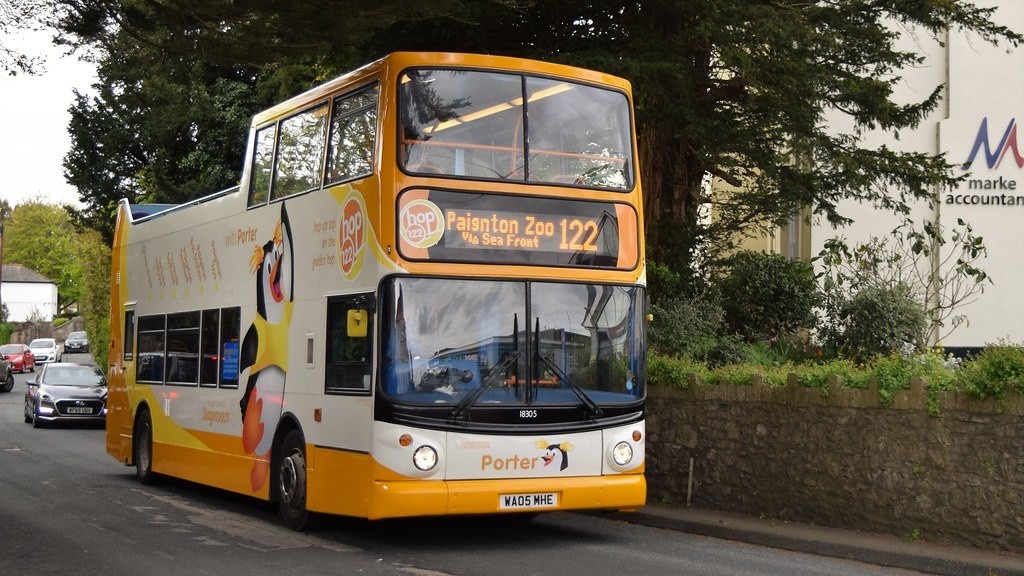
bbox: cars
[0,343,36,373]
[0,352,15,393]
[24,362,108,429]
[64,331,92,352]
[28,337,61,363]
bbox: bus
[105,49,655,533]
